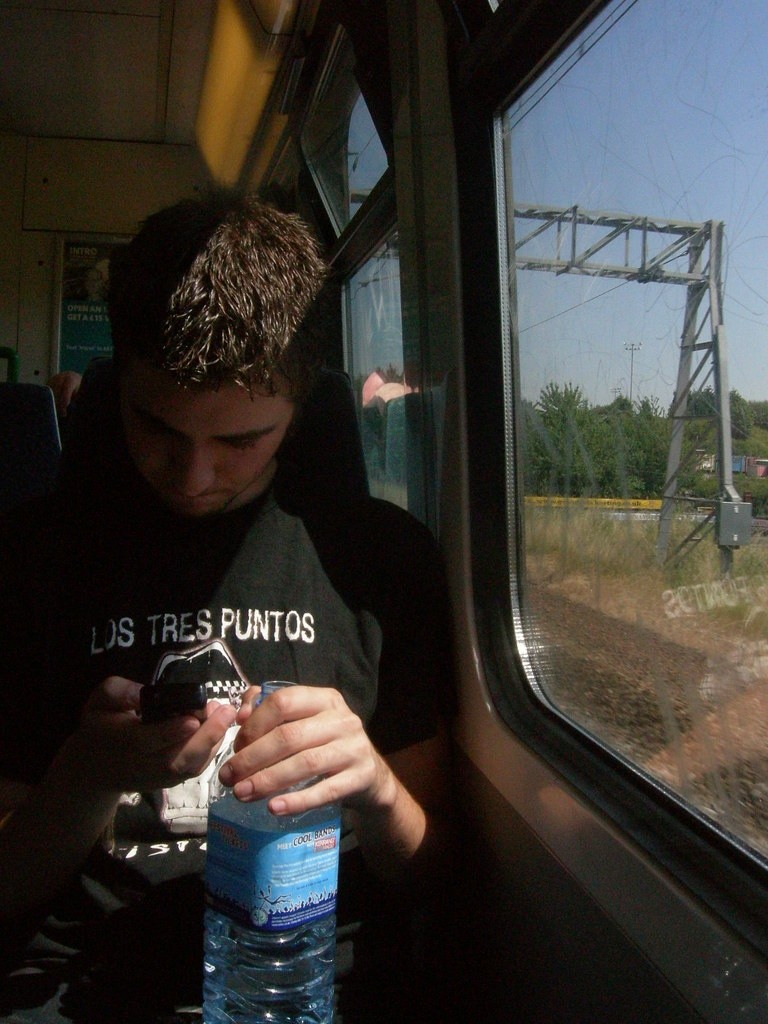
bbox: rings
[50,383,62,390]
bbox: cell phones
[139,681,208,728]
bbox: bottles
[202,680,343,1024]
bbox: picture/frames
[49,232,131,378]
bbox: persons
[45,370,82,416]
[0,185,459,1024]
